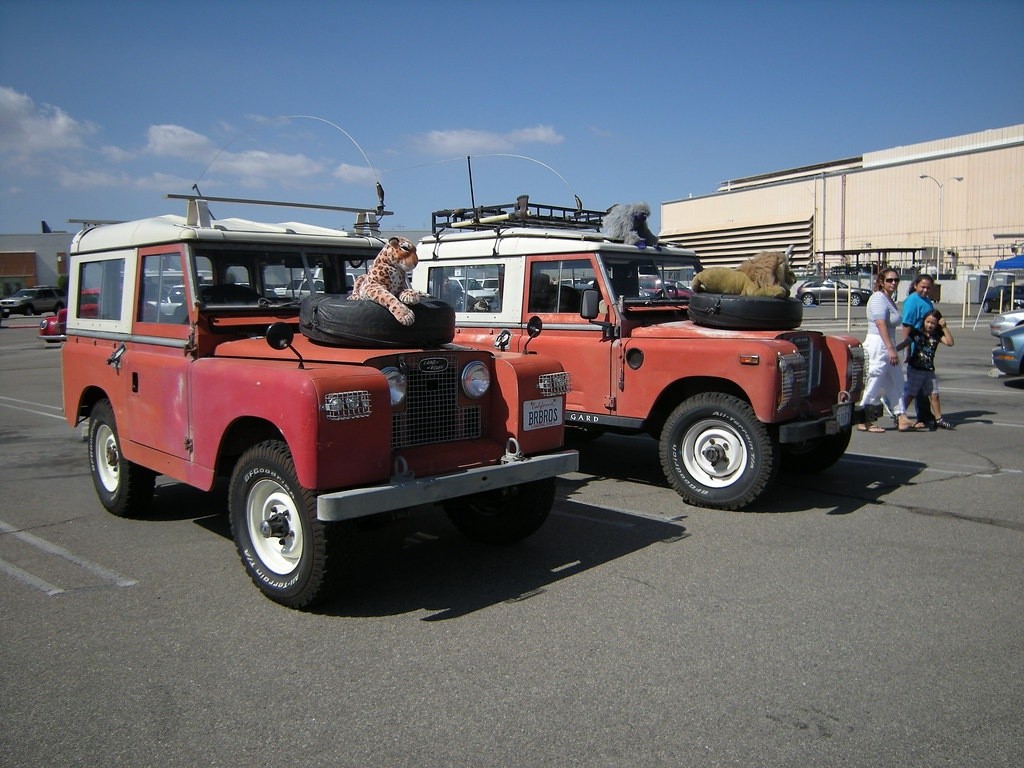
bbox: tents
[973,254,1024,332]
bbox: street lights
[920,174,964,280]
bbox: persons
[856,269,927,433]
[901,274,935,425]
[442,276,456,305]
[895,310,957,430]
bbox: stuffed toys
[690,252,799,298]
[602,201,660,249]
[346,235,420,325]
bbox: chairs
[532,273,578,314]
[200,282,260,305]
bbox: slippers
[858,423,885,433]
[900,421,926,432]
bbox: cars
[983,284,1024,313]
[116,265,690,317]
[992,322,1024,377]
[990,308,1024,338]
[39,287,101,345]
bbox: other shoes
[917,416,938,427]
[880,395,895,418]
[936,419,956,429]
[893,413,901,426]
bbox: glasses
[885,278,900,283]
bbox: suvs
[0,284,67,319]
[62,193,580,611]
[409,194,865,513]
[796,278,873,307]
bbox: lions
[690,251,797,298]
